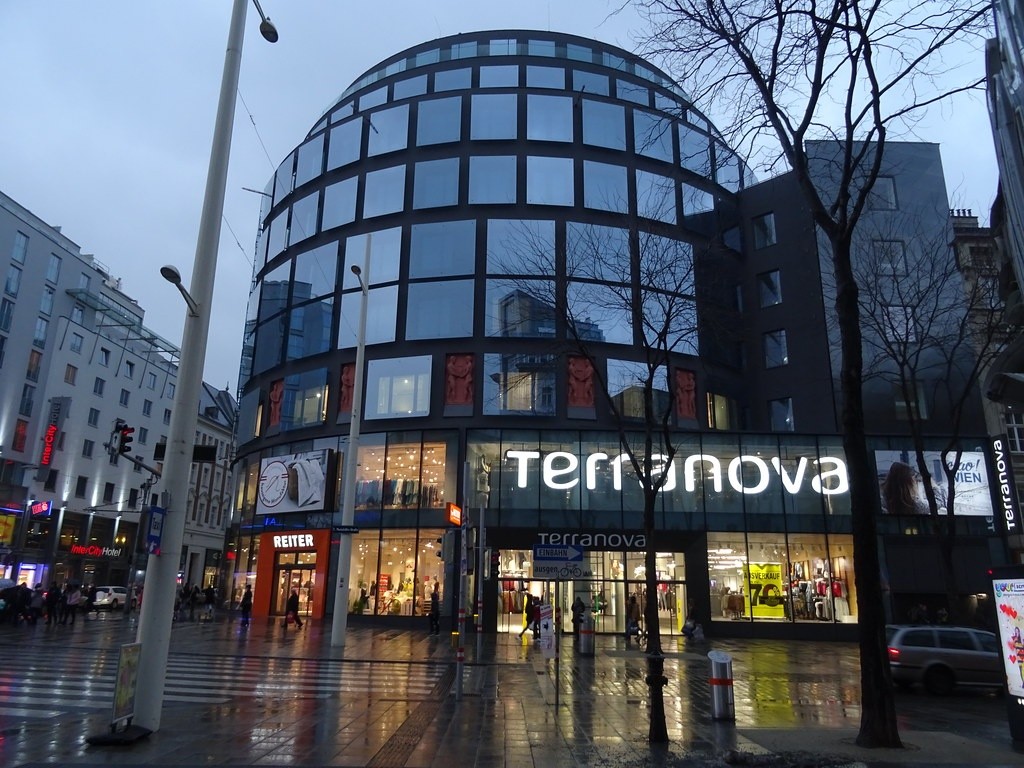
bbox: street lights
[108,261,214,732]
[329,264,372,646]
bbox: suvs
[90,586,138,610]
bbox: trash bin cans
[707,648,738,721]
[578,621,596,655]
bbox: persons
[135,585,144,628]
[370,580,376,595]
[7,580,101,626]
[519,592,546,640]
[446,355,473,404]
[178,583,200,621]
[353,582,370,615]
[414,575,432,601]
[280,588,303,628]
[269,381,283,426]
[572,596,585,634]
[239,585,252,627]
[681,598,699,641]
[432,574,440,600]
[427,593,441,637]
[880,461,930,514]
[341,366,354,411]
[624,596,648,646]
[1012,626,1024,688]
[719,587,730,600]
[676,370,696,418]
[568,356,595,406]
[204,584,216,616]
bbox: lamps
[118,511,123,517]
[30,494,35,500]
[62,501,68,508]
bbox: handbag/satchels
[692,623,705,640]
[629,622,638,635]
[681,618,696,639]
[286,612,295,623]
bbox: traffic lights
[108,414,135,456]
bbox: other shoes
[435,634,441,636]
[519,634,522,640]
[428,633,435,636]
[298,623,303,628]
[45,620,74,625]
[281,625,287,628]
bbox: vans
[883,623,1007,702]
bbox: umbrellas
[0,578,16,589]
